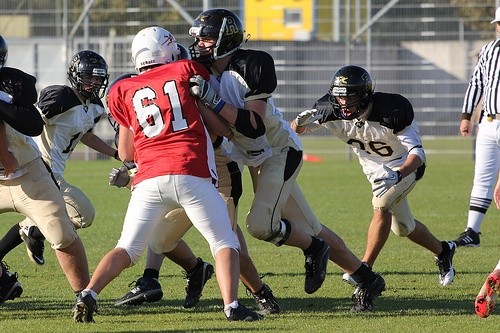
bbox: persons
[0,50,121,300]
[107,73,213,311]
[448,6,500,246]
[290,66,457,287]
[475,172,500,317]
[73,27,265,322]
[149,44,279,315]
[188,8,386,311]
[0,34,91,307]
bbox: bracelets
[114,151,118,158]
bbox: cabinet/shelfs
[73,44,467,136]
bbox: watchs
[392,167,402,184]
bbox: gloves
[108,166,131,188]
[190,74,222,108]
[296,108,322,127]
[371,163,402,197]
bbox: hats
[490,7,500,24]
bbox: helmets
[131,26,178,71]
[0,35,7,69]
[68,51,109,100]
[328,64,373,120]
[187,8,245,67]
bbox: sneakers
[475,270,500,318]
[223,301,264,322]
[304,238,330,294]
[342,273,358,285]
[243,282,279,314]
[0,271,23,305]
[73,291,98,323]
[434,240,457,286]
[19,225,45,265]
[452,226,481,246]
[182,257,215,308]
[350,273,385,313]
[115,277,163,306]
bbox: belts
[488,113,500,120]
